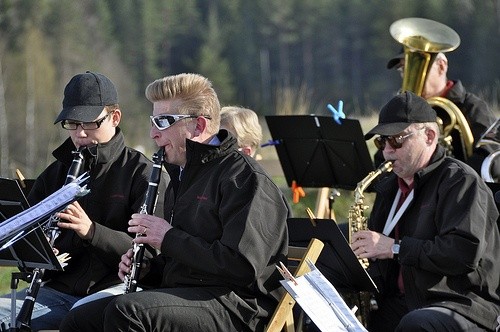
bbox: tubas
[389,16,474,161]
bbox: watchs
[392,238,401,261]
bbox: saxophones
[347,160,394,330]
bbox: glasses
[61,107,117,130]
[374,126,426,150]
[150,115,212,130]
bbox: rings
[143,227,146,233]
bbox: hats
[54,71,118,125]
[387,47,448,70]
[365,91,437,140]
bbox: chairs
[265,238,324,332]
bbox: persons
[70,105,262,311]
[349,88,500,332]
[386,44,500,223]
[0,66,171,332]
[61,73,289,332]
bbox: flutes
[8,146,86,330]
[124,145,165,294]
